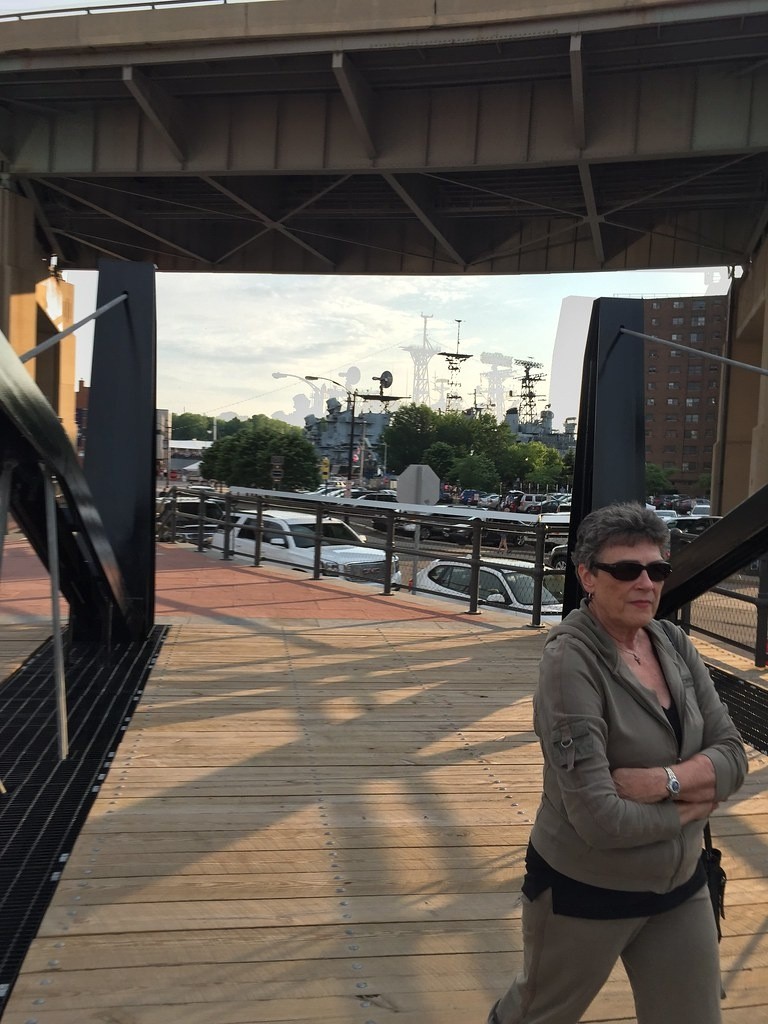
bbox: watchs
[664,766,681,802]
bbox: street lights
[305,375,357,499]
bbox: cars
[295,480,725,572]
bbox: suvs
[210,509,403,591]
[407,553,565,628]
[154,496,224,549]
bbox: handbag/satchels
[702,846,726,944]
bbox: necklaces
[616,631,642,666]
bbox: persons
[487,504,749,1024]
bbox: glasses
[591,560,673,583]
